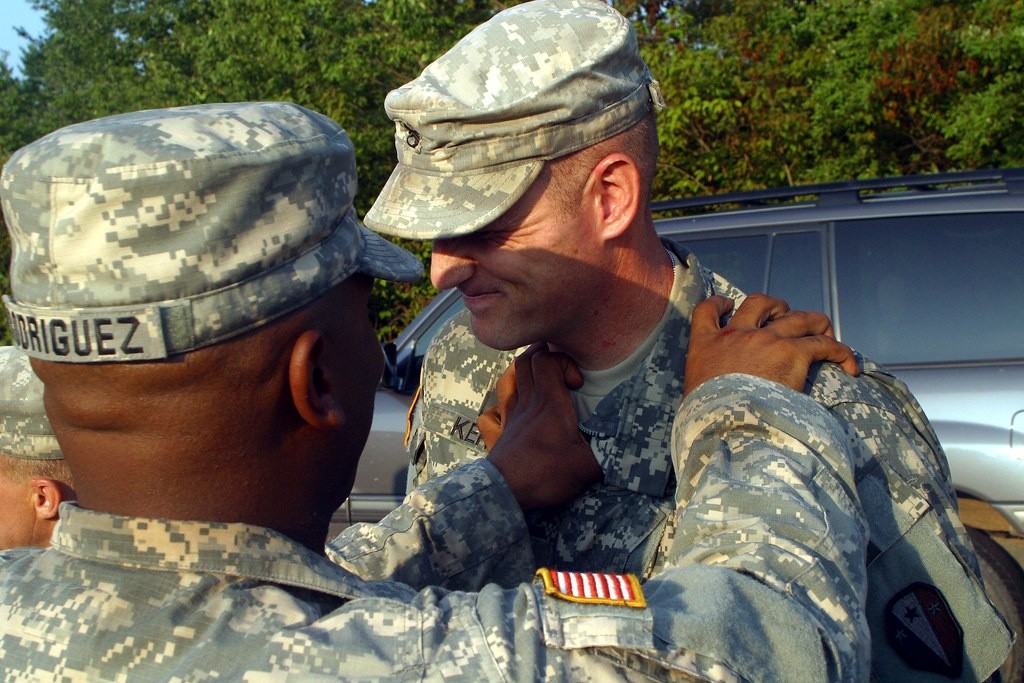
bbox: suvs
[322,170,1024,683]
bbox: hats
[0,346,65,460]
[364,0,666,240]
[0,101,424,363]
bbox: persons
[364,1,1017,683]
[0,102,871,683]
[0,344,77,550]
